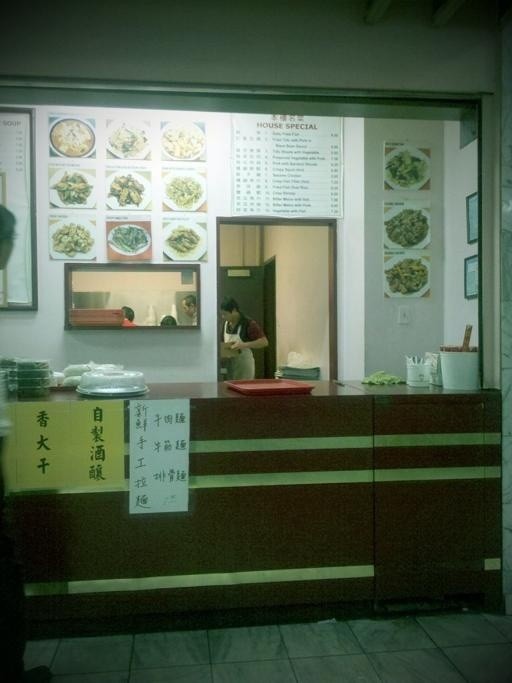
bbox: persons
[159,316,177,325]
[120,306,136,326]
[220,295,269,381]
[183,294,197,327]
[0,205,54,682]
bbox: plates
[48,116,207,261]
[383,146,433,298]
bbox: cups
[408,364,430,388]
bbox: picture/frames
[0,105,38,310]
[463,253,478,300]
[466,191,478,245]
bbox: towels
[362,371,406,385]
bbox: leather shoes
[24,666,52,683]
